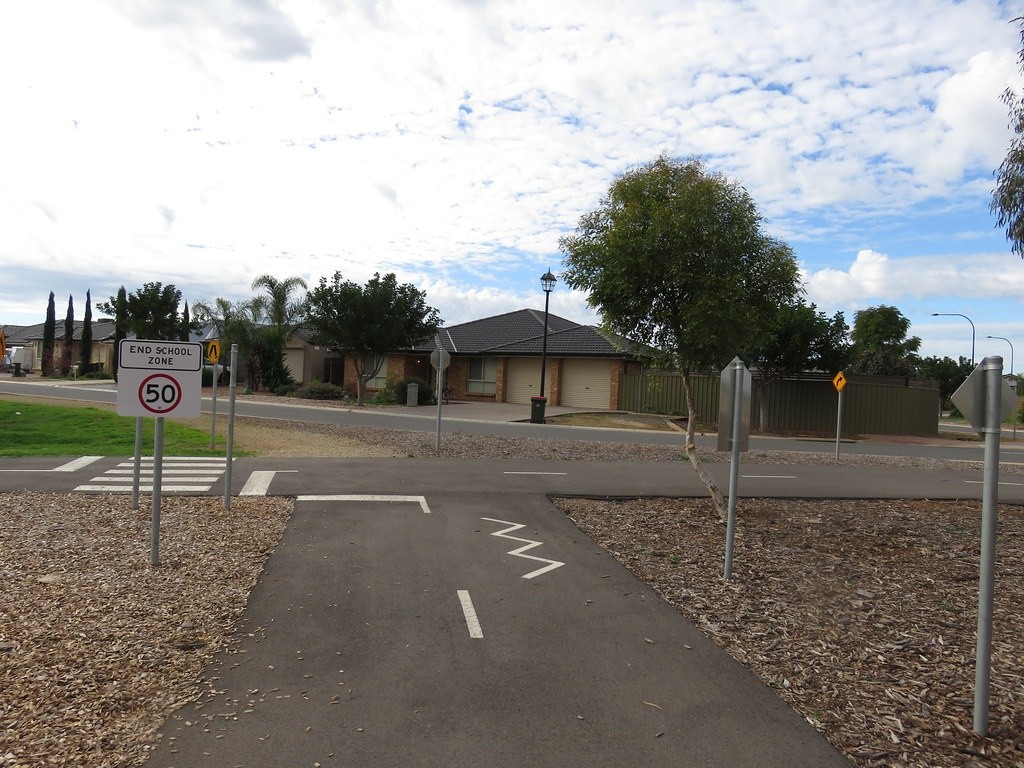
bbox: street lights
[987,335,1014,381]
[930,313,976,369]
[539,267,557,397]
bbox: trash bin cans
[530,396,547,424]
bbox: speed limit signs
[116,338,203,419]
